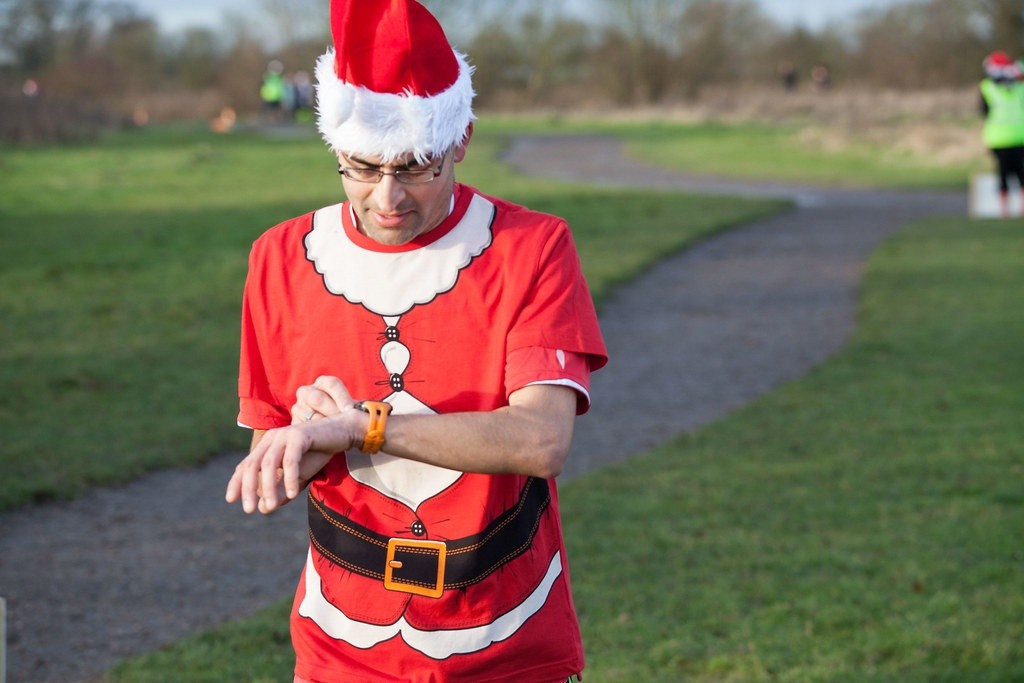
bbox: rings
[306,410,316,420]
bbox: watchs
[354,399,393,456]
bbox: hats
[982,52,1019,80]
[315,0,477,165]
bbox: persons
[222,0,610,682]
[256,56,316,124]
[976,50,1024,218]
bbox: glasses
[338,146,447,185]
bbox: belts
[307,476,552,598]
